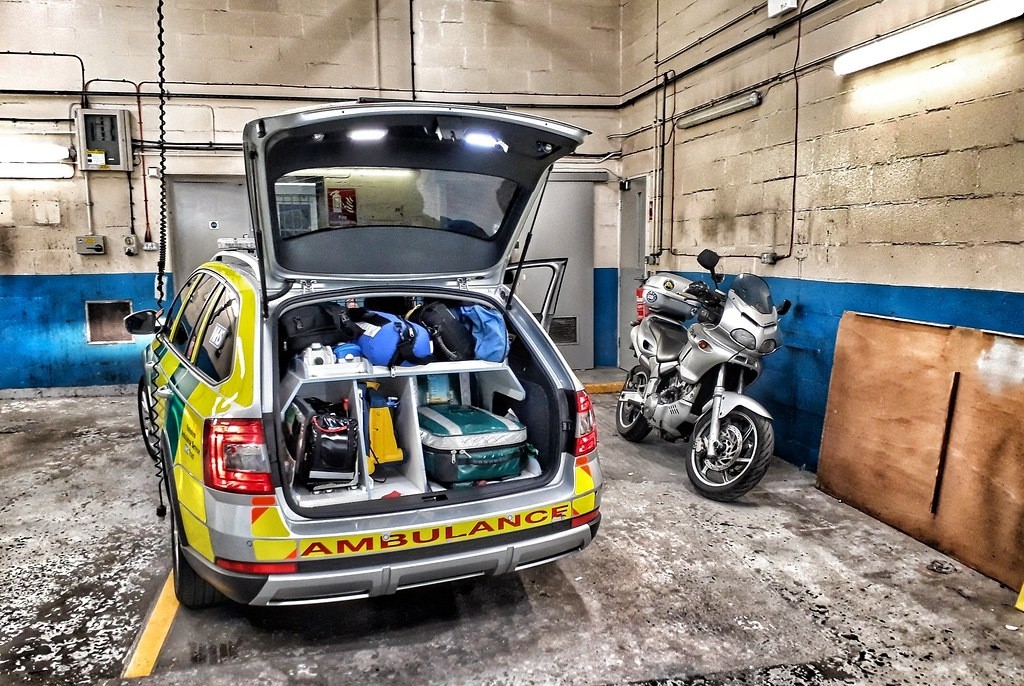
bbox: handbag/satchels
[278,301,511,378]
[303,413,359,492]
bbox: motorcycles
[616,249,792,502]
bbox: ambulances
[124,97,604,608]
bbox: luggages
[417,404,527,489]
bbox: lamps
[832,0,1024,78]
[675,89,763,129]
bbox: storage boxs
[645,273,699,321]
